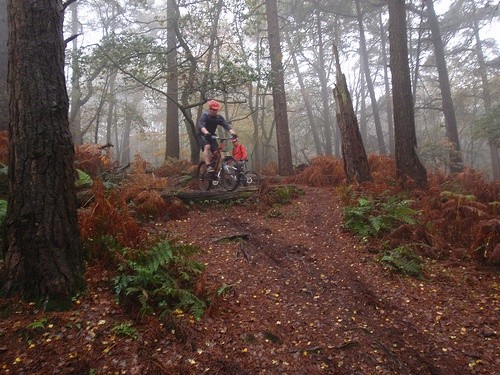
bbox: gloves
[204,133,212,142]
[231,133,238,140]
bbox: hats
[208,100,220,109]
[231,139,237,143]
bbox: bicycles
[218,157,260,187]
[195,135,241,191]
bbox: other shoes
[206,166,215,174]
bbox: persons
[195,99,238,178]
[232,138,248,171]
